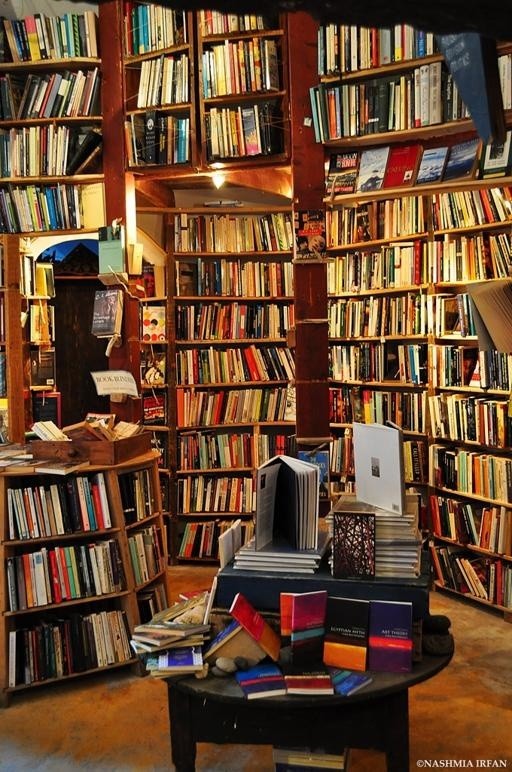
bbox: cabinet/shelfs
[1,244,62,445]
[0,449,172,706]
[0,0,106,234]
[140,207,296,565]
[121,2,292,172]
[312,24,512,620]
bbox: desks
[163,603,454,772]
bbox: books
[186,739,398,769]
[315,204,504,455]
[131,580,435,709]
[11,177,112,230]
[191,554,331,584]
[172,202,301,552]
[6,8,512,195]
[4,465,175,690]
[257,444,324,550]
[315,453,421,571]
[2,234,170,460]
[428,449,509,612]
